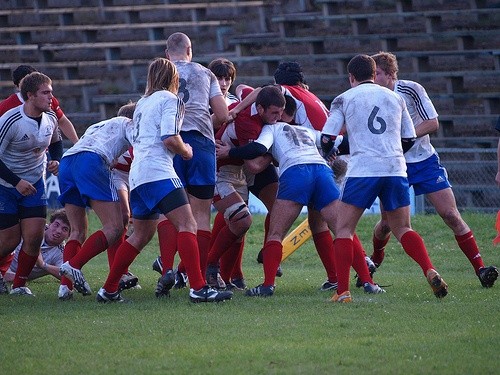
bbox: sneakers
[126,272,141,289]
[319,280,338,292]
[96,287,134,305]
[189,284,233,302]
[246,283,276,296]
[0,272,11,294]
[329,291,351,303]
[58,261,92,296]
[118,274,138,292]
[206,265,247,291]
[58,284,73,300]
[426,269,448,298]
[353,256,376,286]
[9,283,35,297]
[363,283,385,296]
[152,256,163,276]
[154,268,176,300]
[476,266,499,288]
[256,247,282,277]
[172,271,188,289]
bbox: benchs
[1,0,500,212]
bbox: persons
[321,55,448,301]
[356,51,498,288]
[494,138,500,185]
[0,32,386,301]
